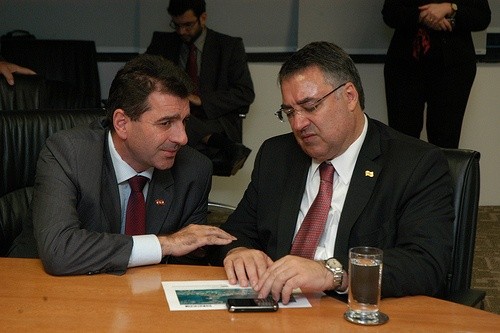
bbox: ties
[187,44,201,93]
[125,175,148,236]
[289,165,336,259]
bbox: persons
[378,0,492,153]
[6,53,239,279]
[198,41,458,307]
[0,61,38,87]
[157,0,255,158]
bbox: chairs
[0,34,104,261]
[431,147,489,313]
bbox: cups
[345,247,383,325]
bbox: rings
[430,16,433,22]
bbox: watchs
[322,257,344,291]
[450,2,458,15]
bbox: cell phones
[226,298,278,312]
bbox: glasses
[275,82,348,123]
[168,15,203,30]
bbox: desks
[1,253,500,333]
